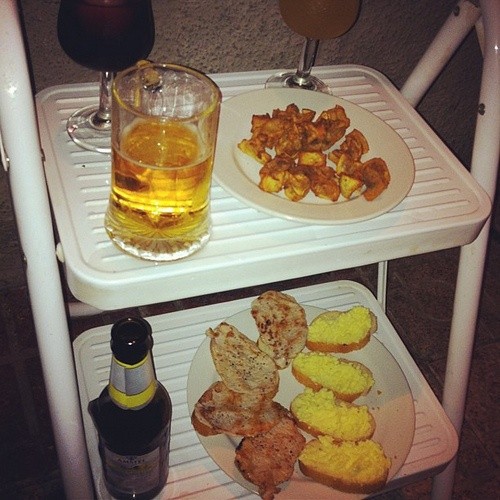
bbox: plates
[209,88,416,224]
[188,306,416,499]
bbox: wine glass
[57,1,155,154]
[265,0,360,98]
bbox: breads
[292,351,374,403]
[250,289,309,369]
[289,393,377,440]
[205,321,281,395]
[299,437,391,493]
[191,381,287,438]
[233,409,306,500]
[304,305,378,353]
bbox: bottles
[95,317,172,500]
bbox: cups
[105,59,222,261]
